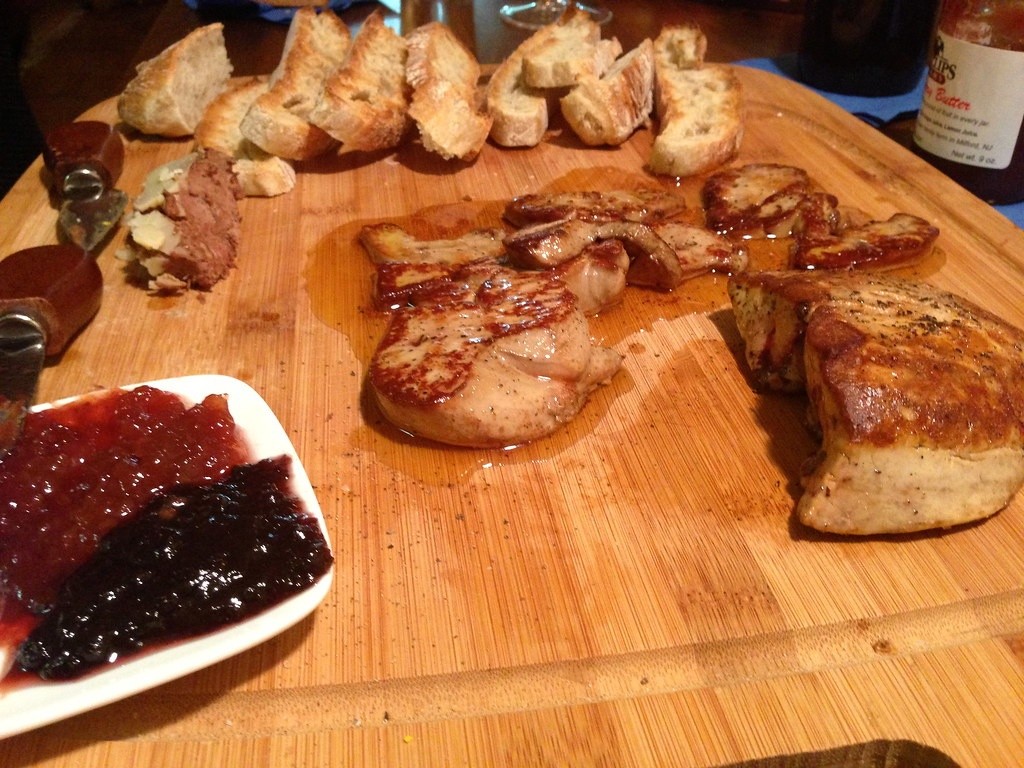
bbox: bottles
[914,1,1024,204]
[799,0,936,97]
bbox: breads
[113,7,744,196]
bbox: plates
[1,375,335,739]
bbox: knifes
[0,338,45,476]
[43,121,129,251]
[1,245,104,359]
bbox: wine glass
[501,0,615,30]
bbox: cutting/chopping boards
[1,62,1024,766]
